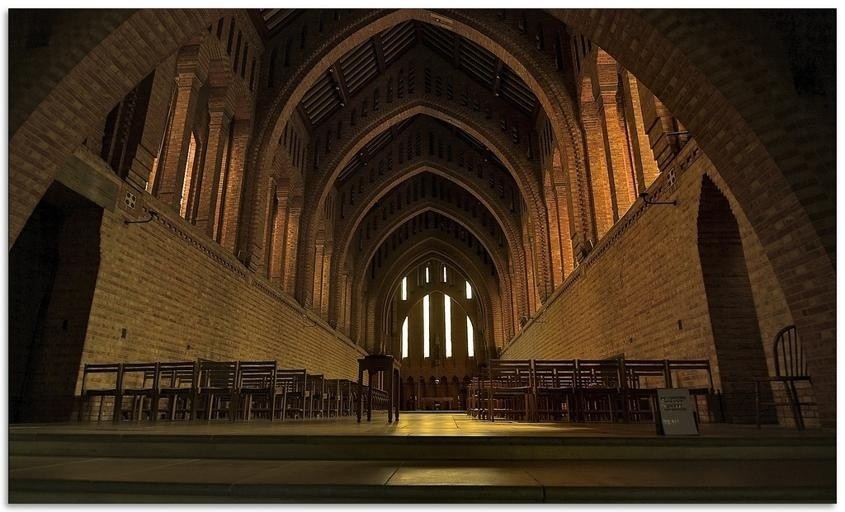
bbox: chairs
[754,324,812,431]
[466,354,722,423]
